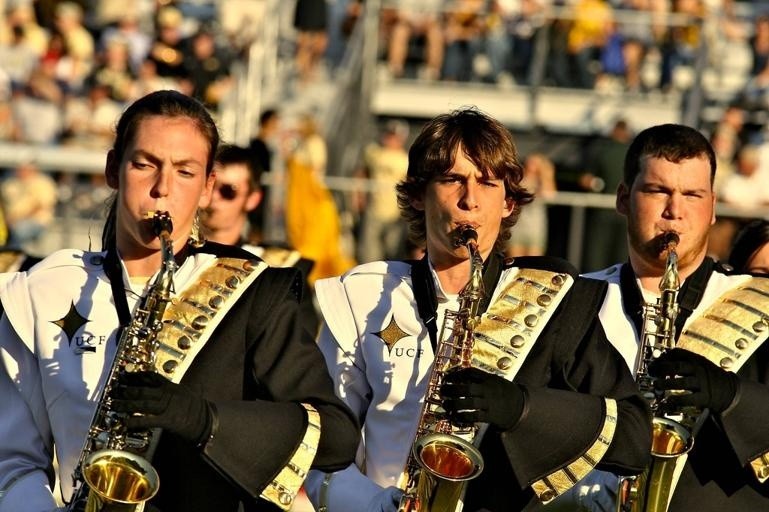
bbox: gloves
[108,369,211,446]
[648,347,738,414]
[440,367,526,431]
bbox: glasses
[208,181,235,201]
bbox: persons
[1,91,358,512]
[1,0,768,340]
[301,111,654,511]
[570,124,768,511]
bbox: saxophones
[618,229,694,512]
[399,224,485,512]
[67,209,179,512]
